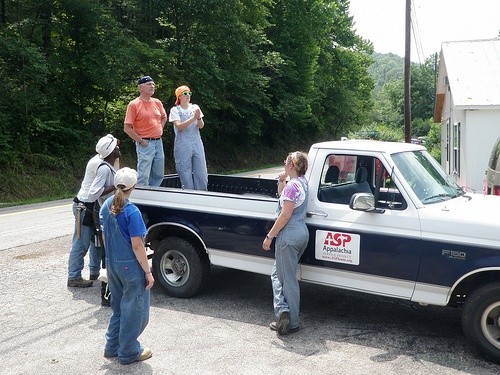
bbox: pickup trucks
[328,154,390,188]
[128,140,500,364]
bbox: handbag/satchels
[101,281,112,306]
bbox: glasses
[181,92,192,96]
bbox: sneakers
[135,346,153,361]
[90,273,99,280]
[68,277,93,287]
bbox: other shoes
[269,321,299,332]
[277,311,290,336]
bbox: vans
[483,136,500,195]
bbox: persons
[123,76,167,187]
[263,150,309,336]
[168,85,208,192]
[99,167,155,364]
[88,134,122,306]
[66,155,119,288]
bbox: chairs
[320,165,372,205]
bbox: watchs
[266,236,273,240]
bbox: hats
[136,75,153,87]
[114,167,138,190]
[175,86,190,105]
[96,134,117,158]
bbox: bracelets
[197,117,202,120]
[145,271,151,274]
[138,139,143,144]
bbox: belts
[142,137,160,140]
